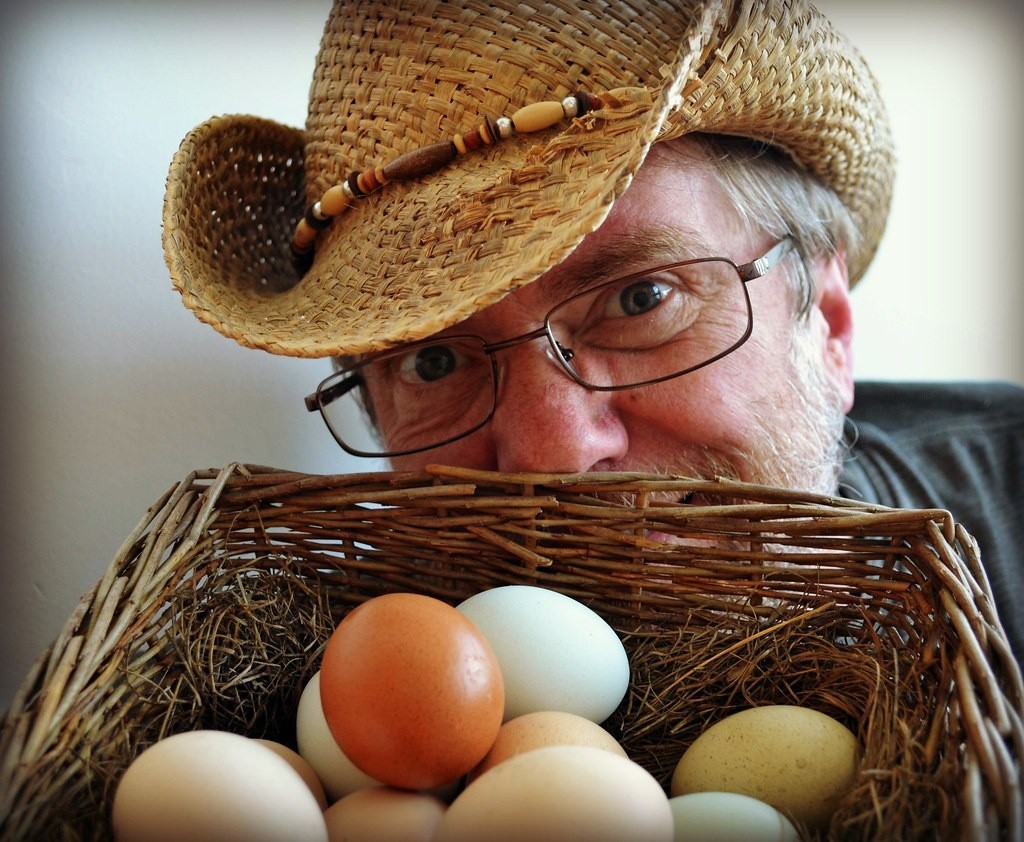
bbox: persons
[162,0,1024,674]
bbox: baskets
[0,461,1024,842]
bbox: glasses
[303,232,799,458]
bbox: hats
[161,0,898,359]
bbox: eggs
[112,584,858,842]
